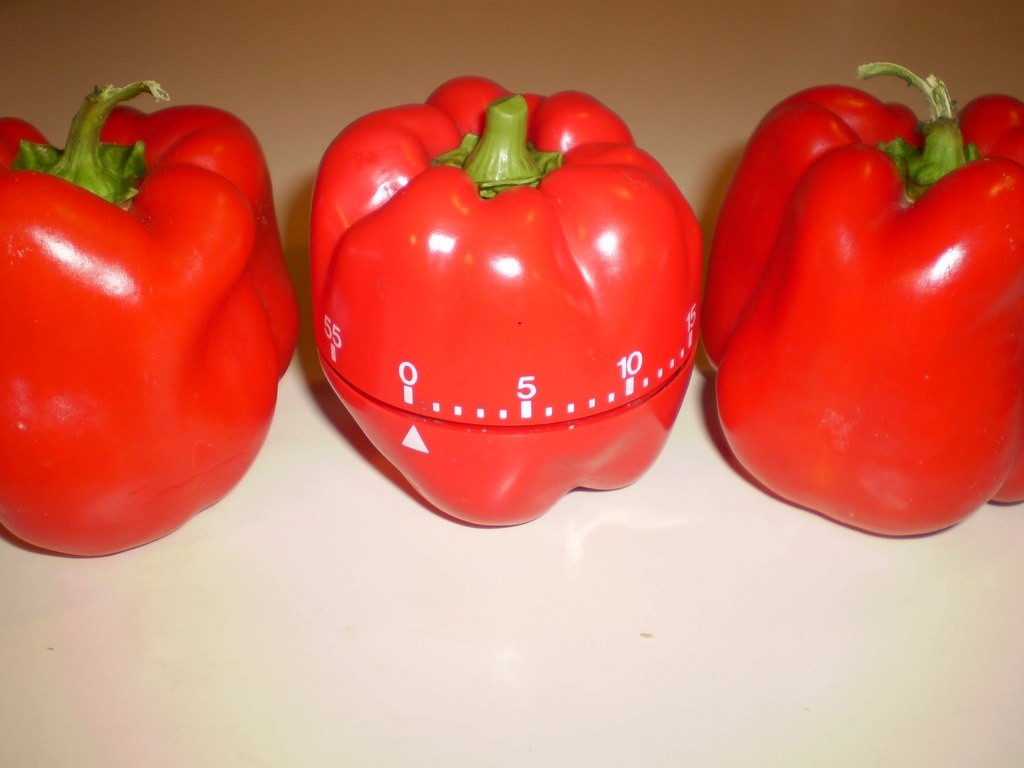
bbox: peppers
[309,75,703,527]
[1,79,301,555]
[703,60,1024,536]
